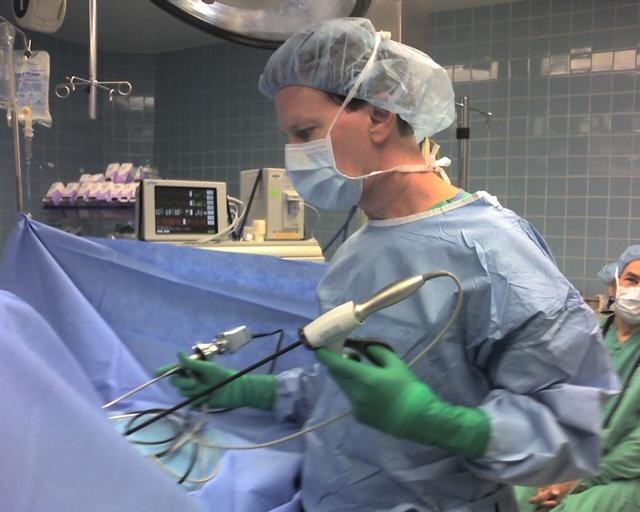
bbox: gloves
[314,343,489,461]
[151,350,276,413]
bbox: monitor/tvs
[136,177,226,242]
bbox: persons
[500,239,640,512]
[150,15,625,512]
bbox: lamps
[149,0,372,51]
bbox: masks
[613,277,640,327]
[282,97,417,212]
